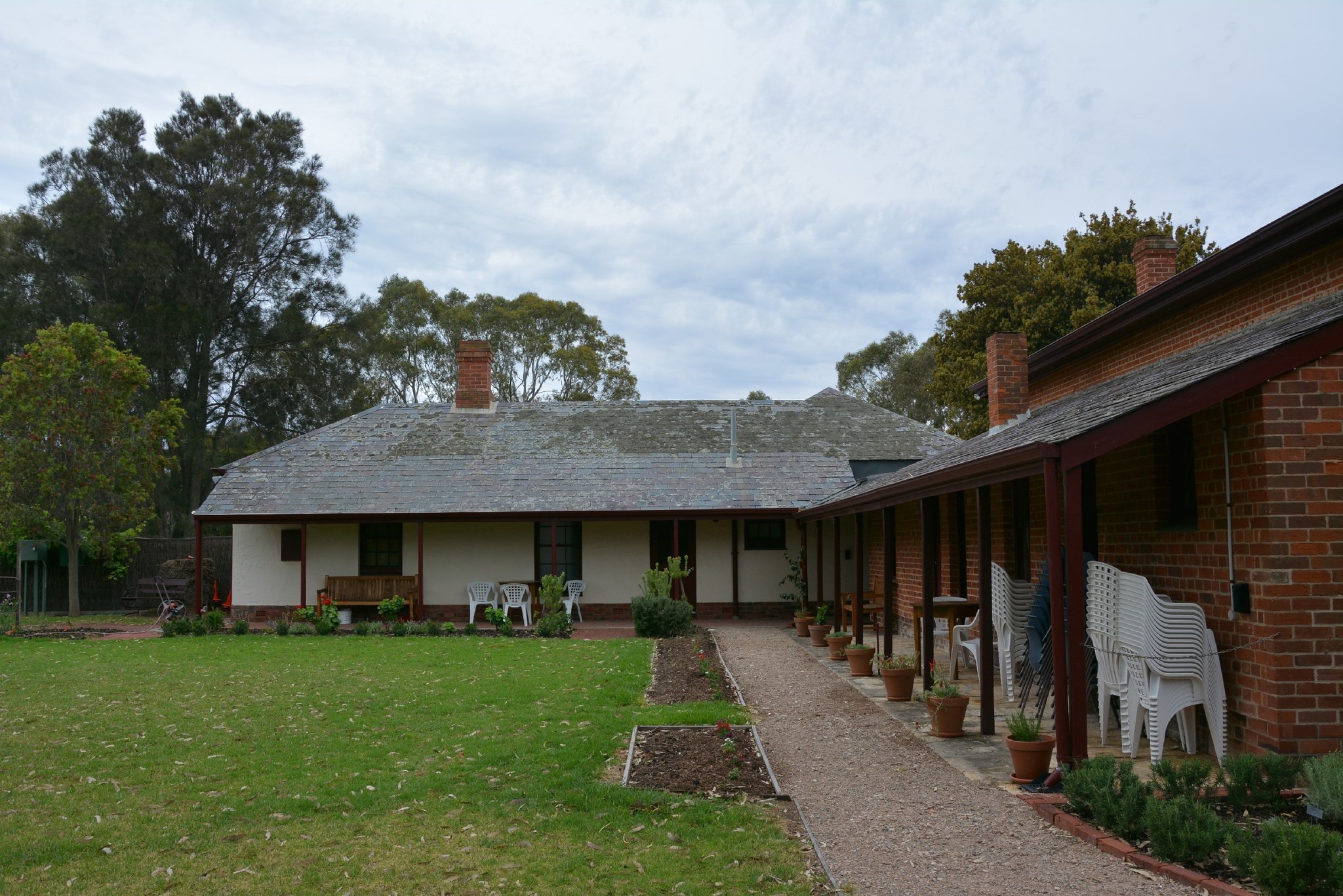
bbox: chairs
[559,580,586,622]
[921,547,1229,770]
[500,584,532,627]
[468,581,498,625]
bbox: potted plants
[1001,708,1056,782]
[808,604,832,646]
[834,642,876,676]
[824,631,852,659]
[778,546,815,636]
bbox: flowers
[911,658,968,736]
[869,650,920,671]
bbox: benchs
[839,575,896,635]
[317,574,418,622]
[121,577,188,614]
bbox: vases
[925,694,969,736]
[879,666,914,701]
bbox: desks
[498,580,542,624]
[909,600,980,680]
